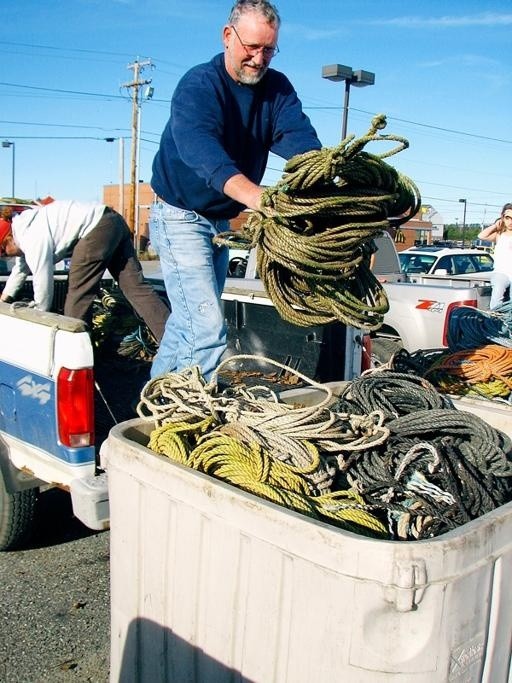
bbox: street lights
[2,139,17,203]
[321,63,378,150]
[459,198,467,249]
[104,135,125,230]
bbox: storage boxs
[97,379,512,683]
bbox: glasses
[231,26,279,58]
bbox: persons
[0,199,171,348]
[475,202,511,309]
[147,0,322,384]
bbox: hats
[0,218,11,256]
